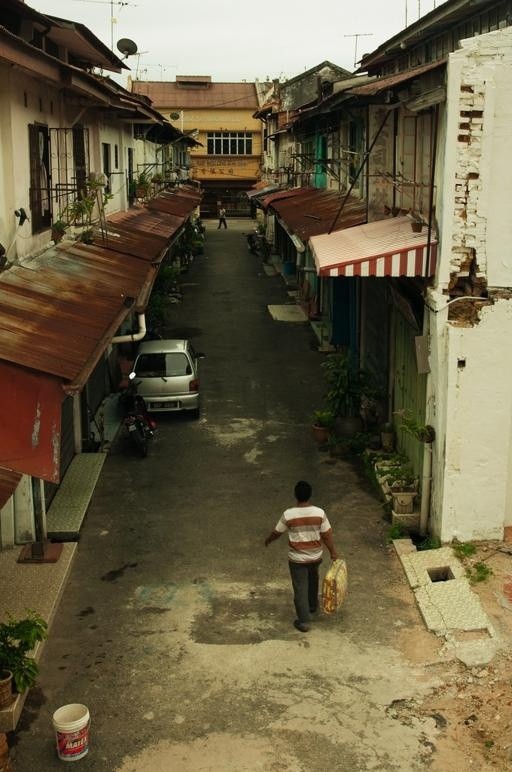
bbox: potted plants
[310,345,421,514]
[134,172,153,197]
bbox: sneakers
[293,619,310,631]
[310,599,319,611]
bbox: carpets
[119,360,134,386]
[269,305,310,323]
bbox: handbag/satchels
[322,558,348,614]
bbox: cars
[134,340,207,420]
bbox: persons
[216,205,228,230]
[263,480,339,634]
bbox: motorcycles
[116,371,158,460]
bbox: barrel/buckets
[53,703,91,761]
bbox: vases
[0,669,14,707]
